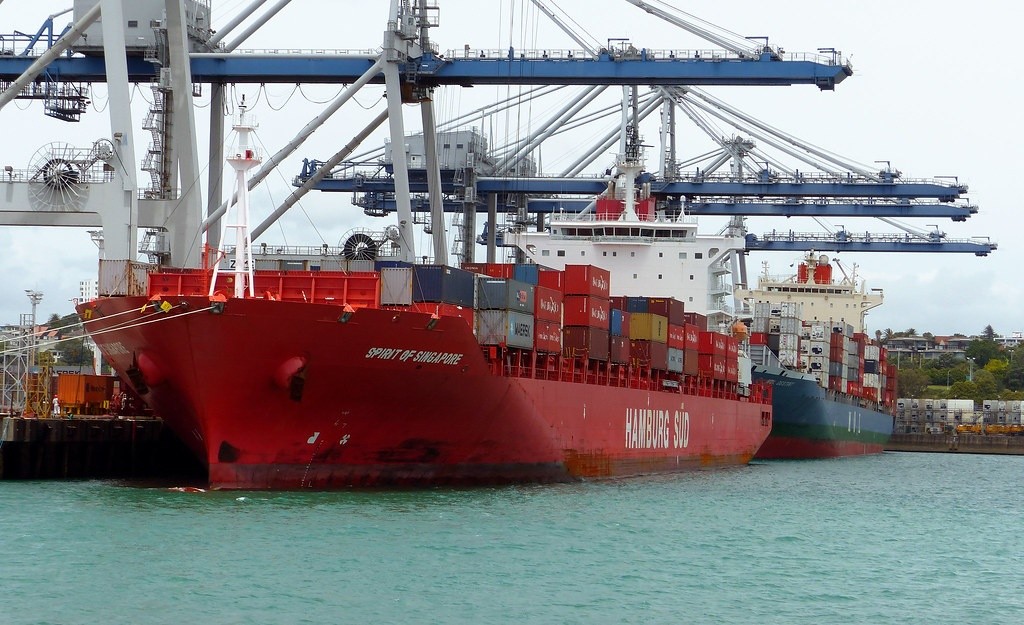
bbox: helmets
[54,395,57,397]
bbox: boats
[0,0,1009,495]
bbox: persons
[52,394,60,414]
